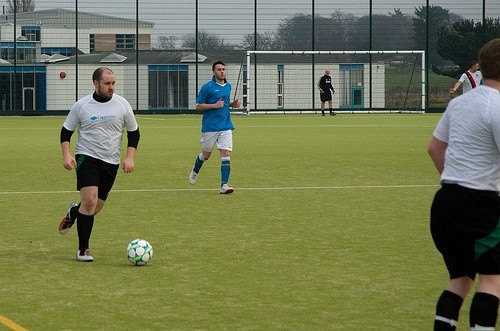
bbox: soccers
[127,239,152,266]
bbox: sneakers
[189,165,199,185]
[219,183,234,194]
[76,249,94,262]
[58,201,78,235]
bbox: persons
[189,60,240,194]
[449,60,483,95]
[58,67,141,262]
[427,36,500,331]
[317,69,336,116]
